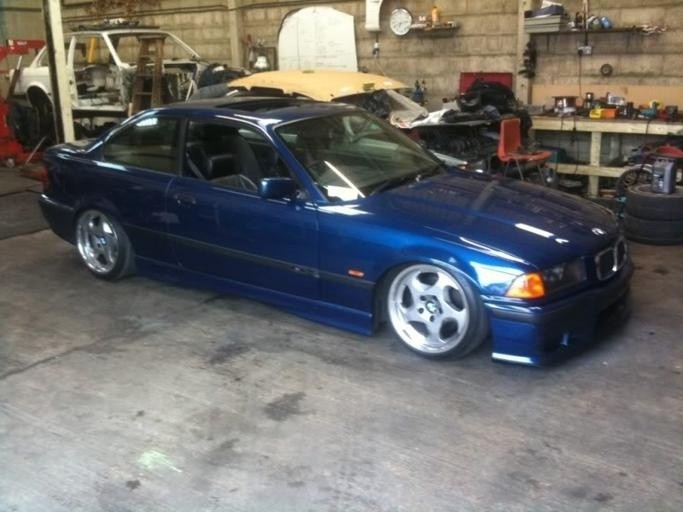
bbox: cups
[625,102,633,120]
[665,106,678,122]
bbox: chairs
[205,153,258,194]
[494,115,555,188]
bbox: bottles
[430,6,439,23]
[586,93,592,109]
[588,14,611,30]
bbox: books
[524,15,570,33]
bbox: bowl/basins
[550,95,578,108]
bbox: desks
[518,111,682,200]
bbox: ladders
[131,33,169,116]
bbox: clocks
[388,6,413,37]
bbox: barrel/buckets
[651,157,677,194]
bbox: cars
[35,92,634,369]
[3,18,251,145]
[187,67,557,193]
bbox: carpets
[0,189,51,241]
